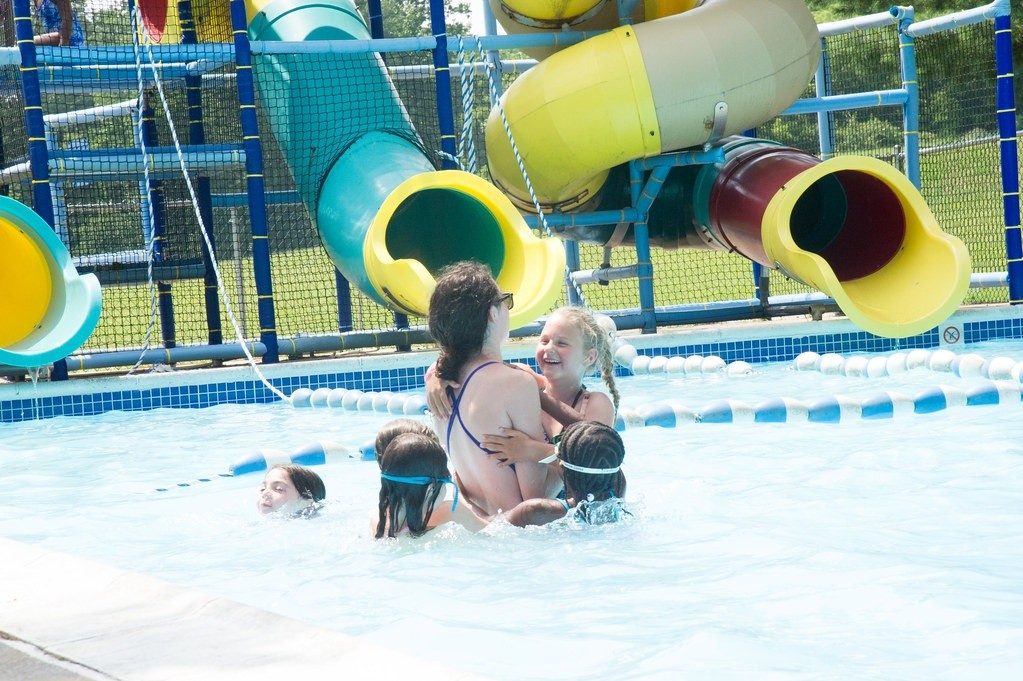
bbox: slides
[1,195,100,365]
[242,0,971,339]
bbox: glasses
[492,292,514,310]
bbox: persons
[423,305,621,500]
[374,418,491,535]
[252,465,325,521]
[374,429,451,539]
[452,420,637,534]
[422,257,569,523]
